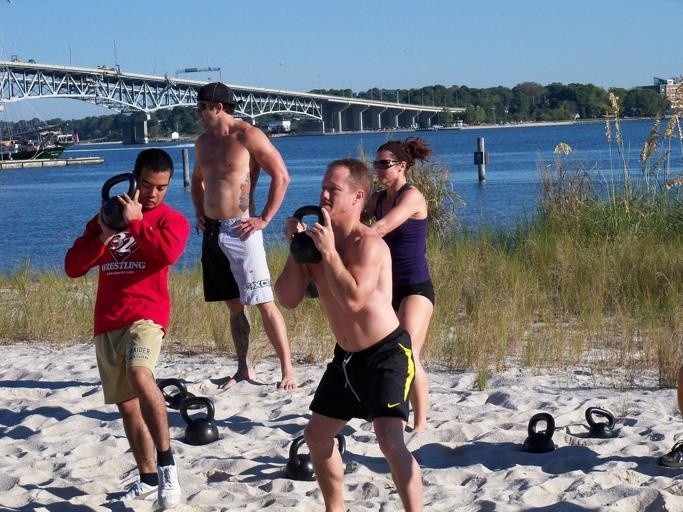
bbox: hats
[197,82,237,104]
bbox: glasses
[372,160,401,169]
[196,102,214,111]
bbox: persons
[273,158,423,511]
[64,148,190,510]
[304,138,436,434]
[191,81,297,392]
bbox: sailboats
[0,47,75,162]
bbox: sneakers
[157,459,181,511]
[122,483,159,502]
[657,443,683,467]
